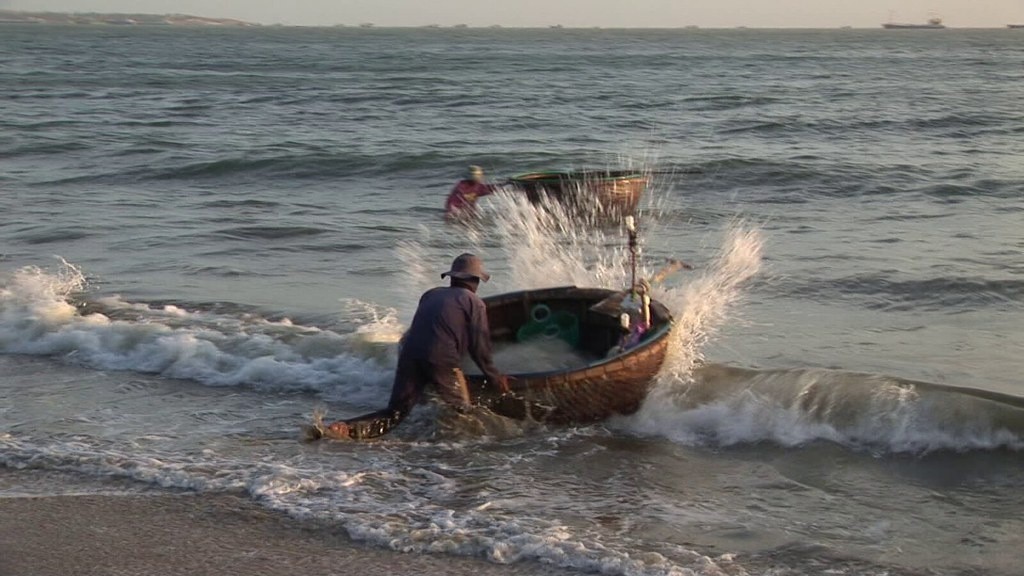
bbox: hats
[441,252,489,284]
[462,165,485,180]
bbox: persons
[441,165,508,226]
[328,254,510,441]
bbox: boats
[506,168,650,222]
[397,285,674,424]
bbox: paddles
[635,166,705,174]
[585,258,693,320]
